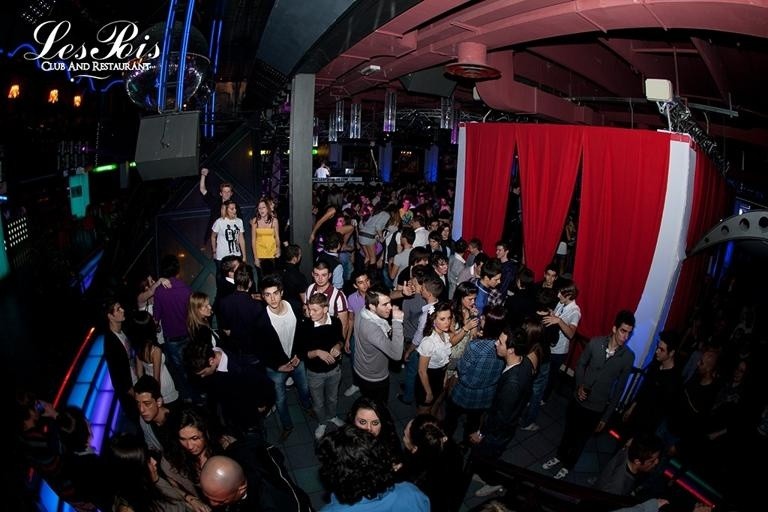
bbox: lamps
[349,103,362,139]
[382,90,398,133]
[328,113,338,142]
[335,99,344,132]
[645,78,731,175]
[450,119,460,145]
[440,98,453,129]
[312,116,319,148]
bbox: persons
[9,159,767,512]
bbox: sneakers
[279,426,295,443]
[343,384,361,397]
[314,423,327,441]
[520,423,539,430]
[471,473,502,497]
[541,457,569,480]
[396,381,414,407]
[328,416,344,427]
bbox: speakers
[400,66,458,98]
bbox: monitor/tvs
[344,168,354,177]
